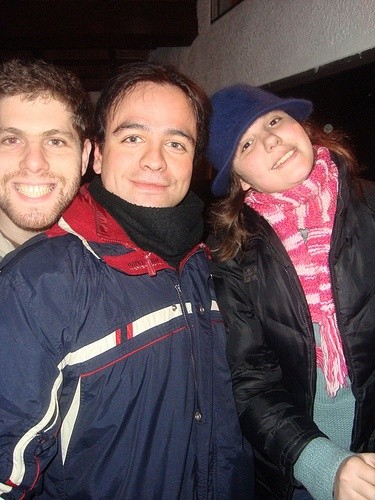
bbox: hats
[206,84,312,197]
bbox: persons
[203,81,375,500]
[0,65,253,499]
[0,59,91,263]
